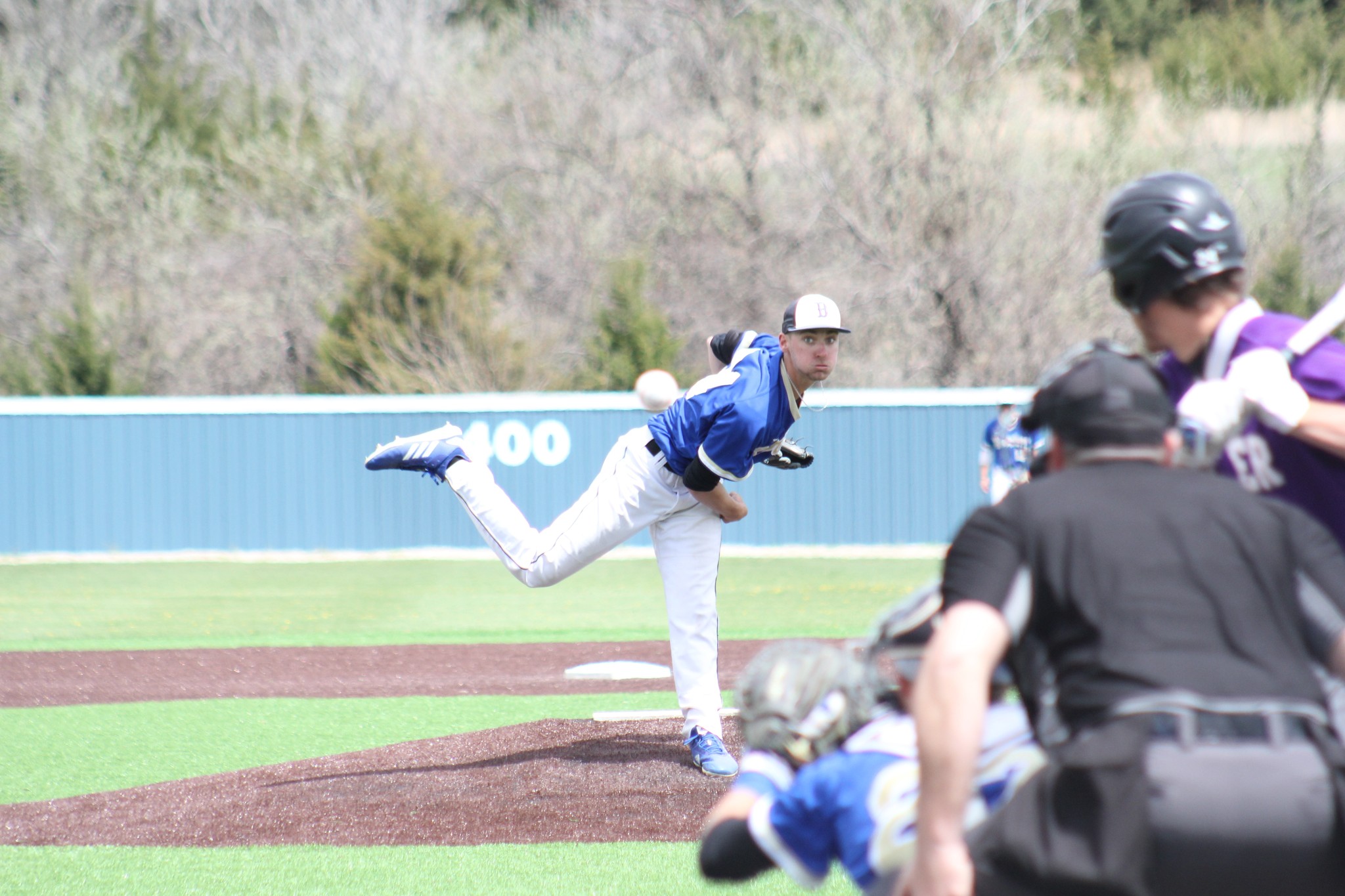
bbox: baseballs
[632,366,680,412]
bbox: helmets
[1102,173,1245,308]
[867,587,1014,684]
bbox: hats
[1020,339,1175,434]
[782,293,851,336]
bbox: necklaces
[781,357,828,412]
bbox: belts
[1102,710,1312,740]
[647,440,681,477]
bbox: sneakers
[689,726,738,777]
[364,425,472,483]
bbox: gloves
[1176,379,1250,463]
[1224,347,1307,433]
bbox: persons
[367,295,851,778]
[699,174,1345,896]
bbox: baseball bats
[1178,283,1345,458]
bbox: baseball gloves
[760,435,815,470]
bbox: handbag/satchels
[969,722,1151,896]
